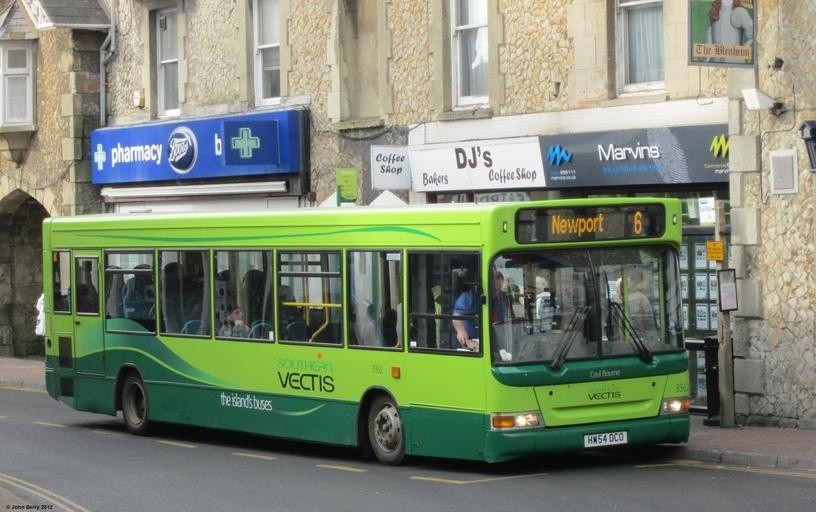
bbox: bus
[42,197,690,465]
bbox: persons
[216,303,252,339]
[701,0,754,64]
[535,276,560,319]
[452,268,512,349]
[614,277,659,342]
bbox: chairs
[182,320,308,342]
